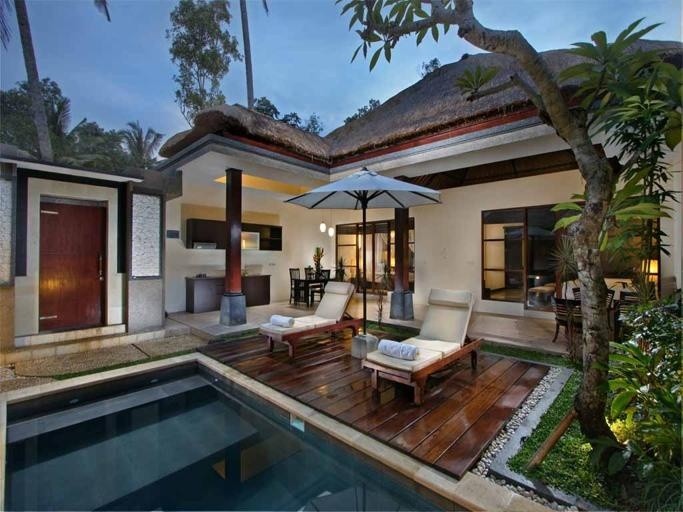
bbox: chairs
[553,284,643,343]
[255,276,359,365]
[287,265,346,307]
[360,286,486,405]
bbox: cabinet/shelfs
[183,273,272,312]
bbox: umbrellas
[283,166,442,335]
